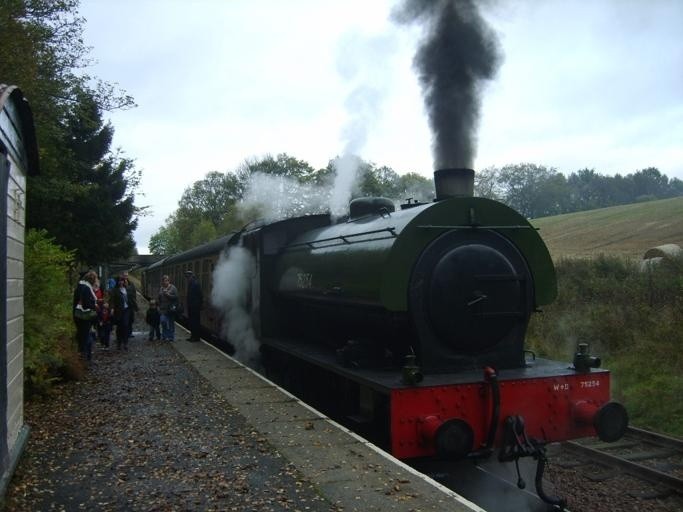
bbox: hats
[119,271,129,279]
[184,271,193,277]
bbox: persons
[156,275,179,344]
[144,299,161,342]
[72,269,139,367]
[184,270,203,343]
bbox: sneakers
[100,334,135,353]
[148,336,177,343]
[186,336,201,342]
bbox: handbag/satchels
[73,306,99,325]
[167,301,185,317]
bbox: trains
[140,167,631,509]
